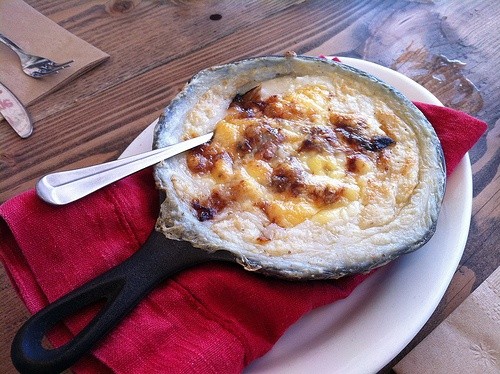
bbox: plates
[110,53,472,374]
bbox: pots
[7,51,452,374]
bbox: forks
[0,33,74,80]
